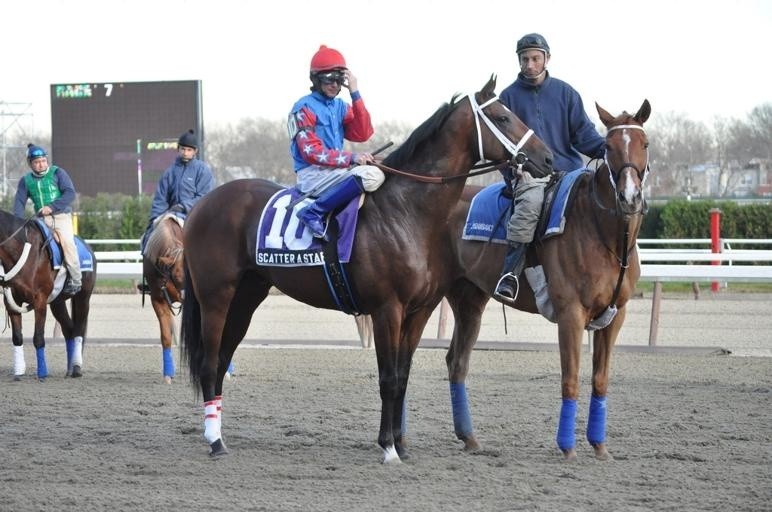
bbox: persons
[496,33,605,301]
[14,143,82,296]
[137,129,214,294]
[288,45,385,242]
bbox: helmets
[516,33,551,85]
[309,48,348,99]
[26,144,48,162]
[178,128,198,148]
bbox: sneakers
[137,283,151,295]
[62,280,82,296]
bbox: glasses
[314,71,347,85]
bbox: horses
[143,210,235,386]
[0,208,97,381]
[401,98,652,465]
[182,74,554,463]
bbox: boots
[495,241,528,299]
[297,175,364,241]
